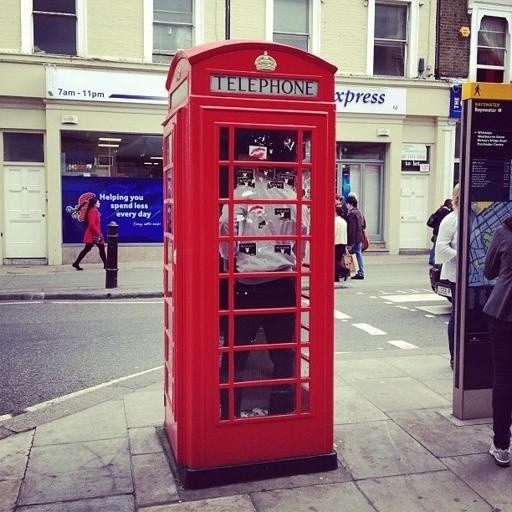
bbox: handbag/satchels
[426,206,441,228]
[361,230,369,252]
[340,249,359,273]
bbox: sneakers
[352,274,364,279]
[488,439,511,466]
[72,262,106,270]
[239,406,268,419]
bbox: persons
[217,135,310,418]
[301,188,308,262]
[427,198,454,266]
[480,214,512,466]
[431,182,460,371]
[334,192,369,282]
[72,197,108,270]
